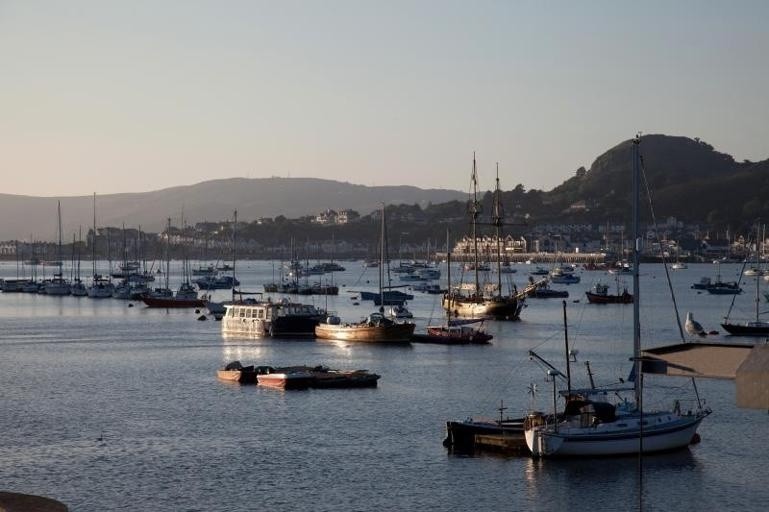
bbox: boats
[706,277,743,295]
[720,217,769,335]
[744,266,769,281]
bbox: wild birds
[684,311,709,342]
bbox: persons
[208,294,212,303]
[267,295,271,303]
[672,400,681,416]
